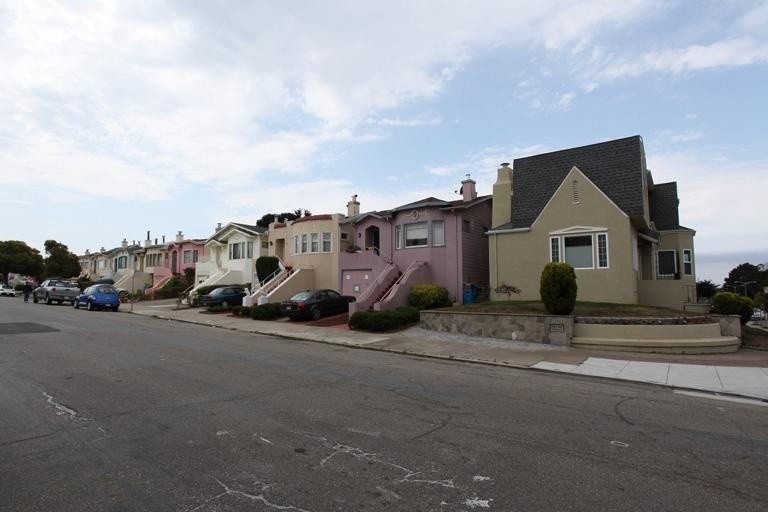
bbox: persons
[23,282,32,301]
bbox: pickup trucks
[32,279,82,306]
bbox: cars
[74,283,121,311]
[279,288,357,323]
[199,286,252,310]
[0,284,17,297]
